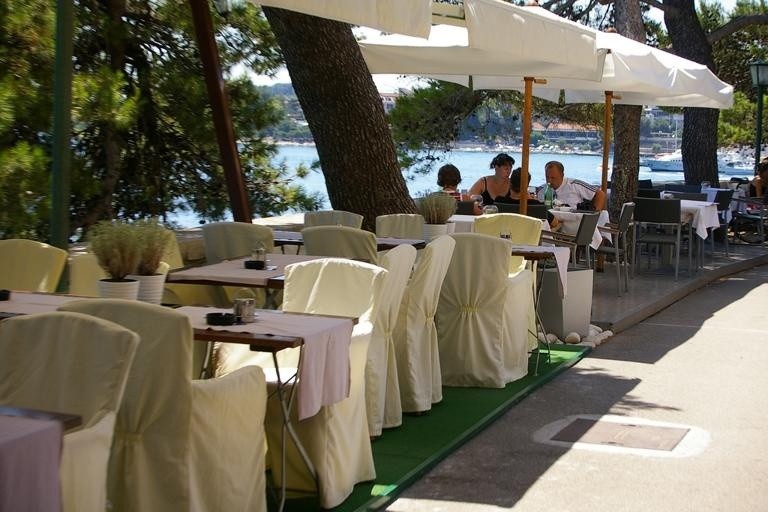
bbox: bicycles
[696,171,768,246]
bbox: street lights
[746,51,768,179]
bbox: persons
[434,162,483,216]
[467,152,513,204]
[532,160,607,211]
[748,162,768,198]
[497,167,558,228]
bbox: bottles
[543,181,554,209]
[244,248,272,269]
[500,233,512,240]
[233,298,255,323]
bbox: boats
[461,140,601,156]
[638,152,655,166]
[646,149,756,177]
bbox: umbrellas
[407,4,659,212]
[247,0,598,69]
[531,28,735,196]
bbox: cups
[457,189,499,214]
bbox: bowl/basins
[554,206,571,211]
[206,313,232,326]
